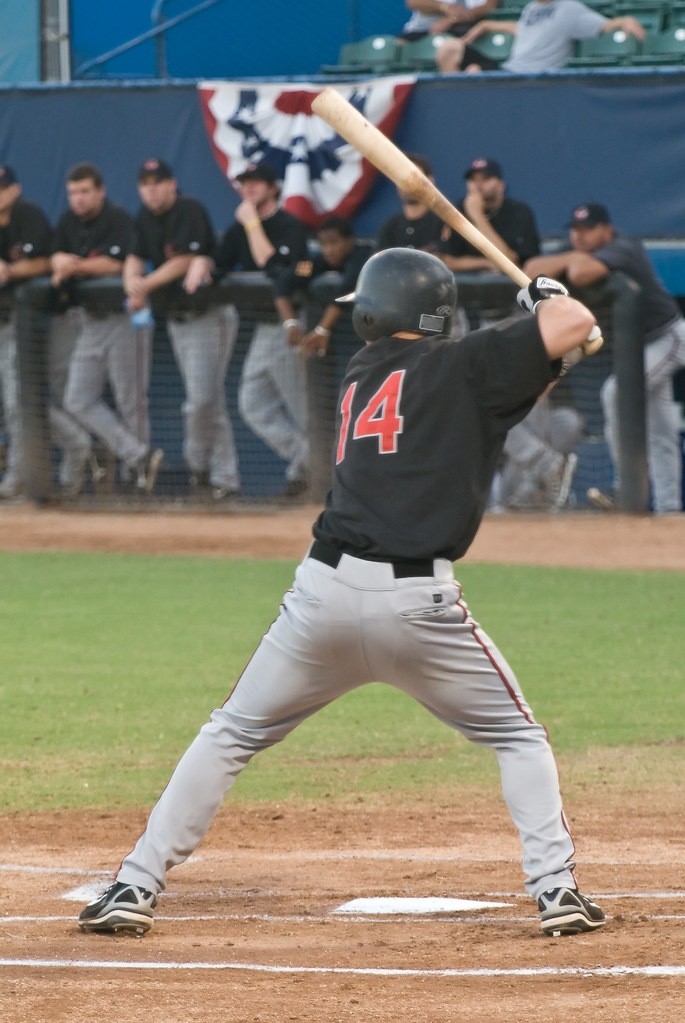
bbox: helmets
[334,247,457,342]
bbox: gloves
[517,274,570,315]
[557,325,602,376]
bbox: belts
[309,541,434,579]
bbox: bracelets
[282,318,304,329]
[314,324,332,338]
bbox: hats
[138,160,173,178]
[466,157,501,180]
[236,162,275,182]
[0,167,13,189]
[563,203,608,228]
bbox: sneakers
[77,882,156,935]
[538,887,607,936]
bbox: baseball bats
[312,86,604,355]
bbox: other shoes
[586,488,619,509]
[137,447,164,493]
[278,480,302,496]
[551,454,576,507]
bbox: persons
[270,217,376,364]
[436,1,649,75]
[397,0,497,45]
[76,249,610,935]
[121,157,242,505]
[0,162,65,508]
[523,200,685,512]
[436,159,577,512]
[48,166,163,498]
[374,149,450,266]
[190,162,323,495]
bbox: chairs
[316,0,685,72]
